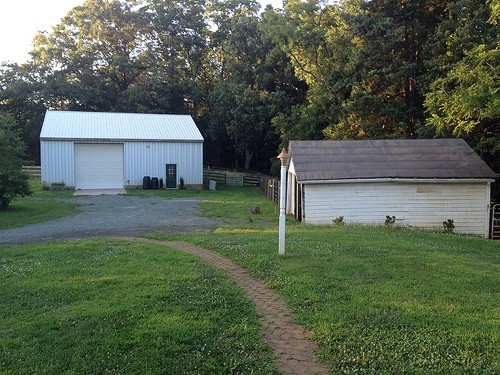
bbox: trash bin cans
[151,177,158,189]
[143,176,150,189]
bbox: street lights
[276,148,291,256]
